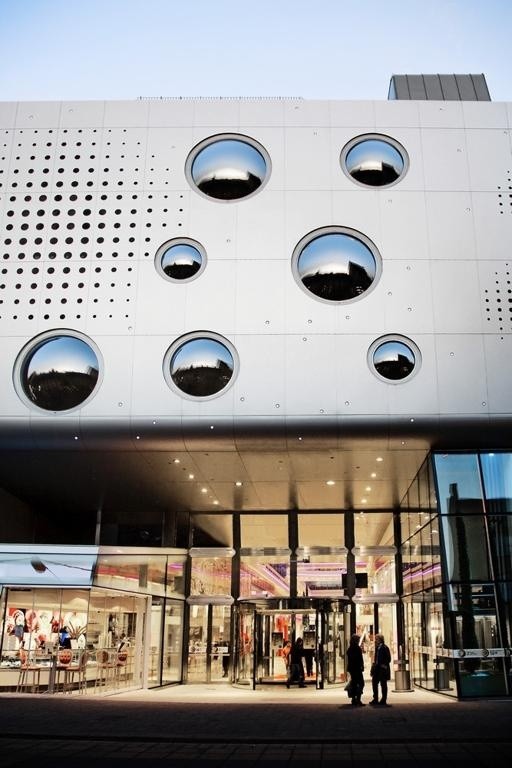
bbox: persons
[195,638,202,644]
[347,633,366,705]
[221,640,231,681]
[303,646,314,677]
[369,633,391,706]
[316,636,320,674]
[119,632,127,643]
[212,641,218,660]
[286,637,307,688]
[58,626,72,649]
[282,641,291,667]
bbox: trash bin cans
[432,669,452,691]
[395,670,410,690]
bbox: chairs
[14,648,130,695]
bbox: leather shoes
[299,685,307,688]
[285,682,289,688]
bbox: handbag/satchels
[345,681,362,696]
[371,663,389,680]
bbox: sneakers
[351,699,386,705]
